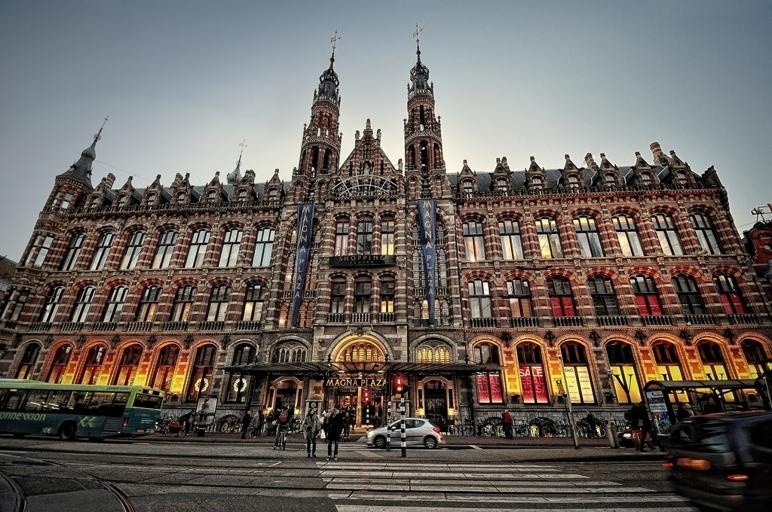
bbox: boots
[306,454,317,458]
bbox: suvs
[664,410,772,512]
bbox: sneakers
[333,456,338,460]
[326,456,330,461]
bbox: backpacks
[279,411,289,424]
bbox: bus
[0,378,163,443]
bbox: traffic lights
[395,377,403,392]
[363,390,370,403]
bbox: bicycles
[271,424,288,450]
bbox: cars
[164,421,183,432]
[365,417,446,451]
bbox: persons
[501,409,511,440]
[241,401,355,464]
